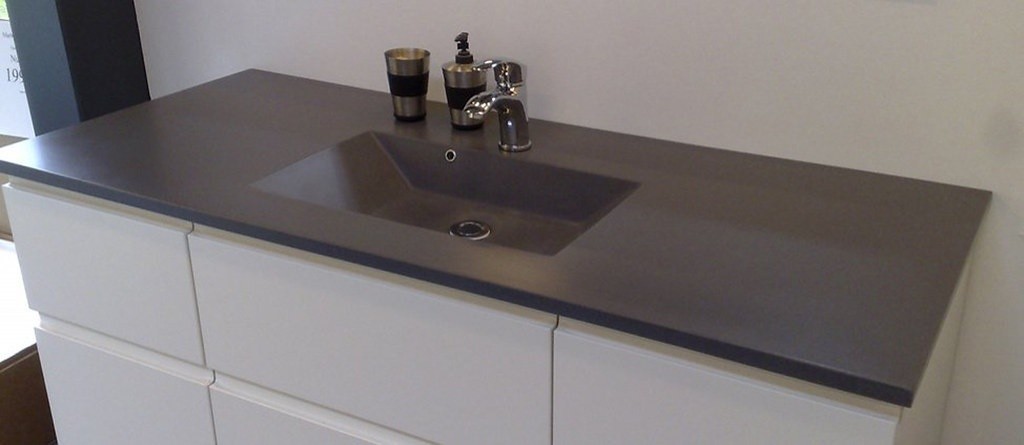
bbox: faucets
[462,59,534,152]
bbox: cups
[384,47,430,122]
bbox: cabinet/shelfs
[0,69,993,445]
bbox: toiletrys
[441,31,487,129]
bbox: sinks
[250,131,643,257]
